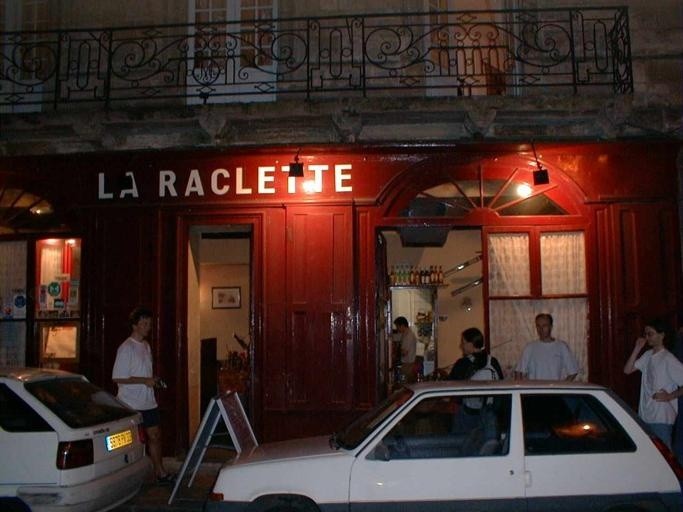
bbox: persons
[514,313,578,383]
[111,306,177,484]
[624,318,683,451]
[442,328,504,435]
[393,317,416,383]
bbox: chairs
[458,407,501,457]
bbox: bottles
[389,263,444,284]
[225,350,247,367]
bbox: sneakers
[155,473,176,485]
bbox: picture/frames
[211,287,241,309]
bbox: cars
[212,380,682,511]
[0,365,146,512]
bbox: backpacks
[464,351,500,408]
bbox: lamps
[287,147,304,177]
[528,138,549,187]
